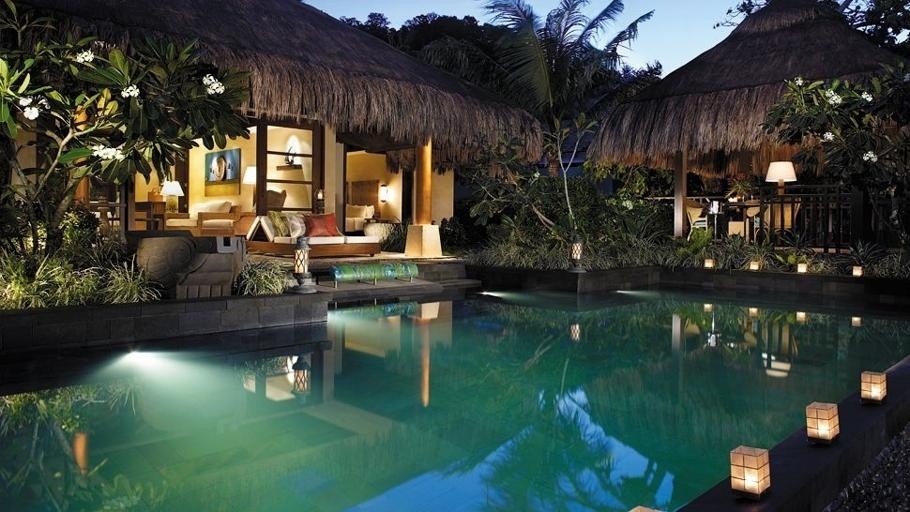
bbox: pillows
[189,201,232,221]
[268,208,337,236]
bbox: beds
[345,204,375,232]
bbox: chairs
[242,215,381,257]
[163,205,241,237]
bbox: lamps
[290,237,318,294]
[290,354,314,407]
[765,161,798,197]
[566,236,587,273]
[625,368,889,512]
[159,180,185,214]
[569,314,581,347]
[242,166,257,185]
